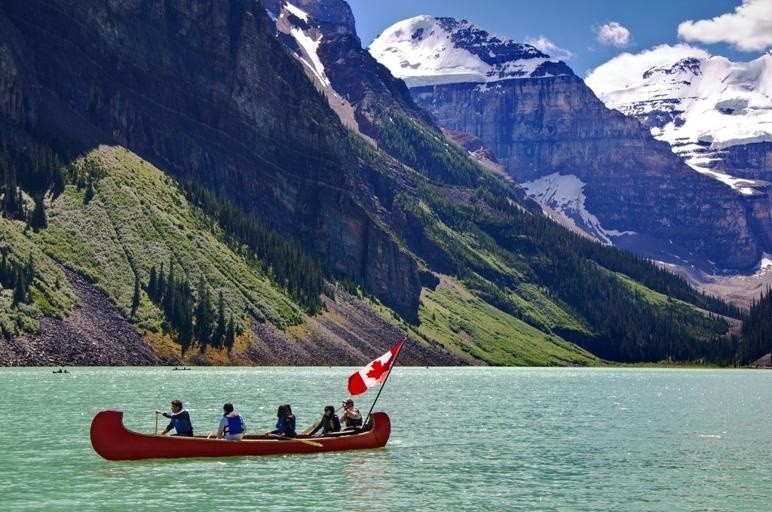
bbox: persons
[339,399,362,426]
[264,405,297,439]
[310,406,341,435]
[217,402,247,440]
[155,400,193,437]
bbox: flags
[348,339,404,396]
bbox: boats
[90,409,391,461]
[53,370,70,374]
[172,368,192,371]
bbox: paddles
[303,406,343,433]
[267,434,323,448]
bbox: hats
[343,399,352,403]
